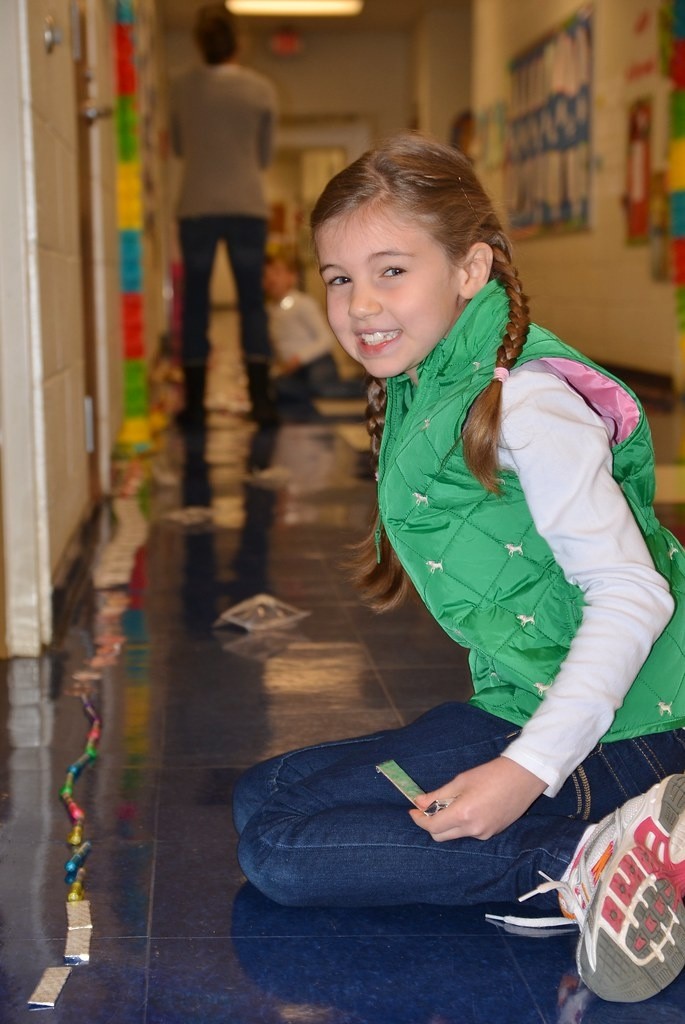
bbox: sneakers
[484,774,685,1002]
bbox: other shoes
[253,379,283,431]
[176,408,206,430]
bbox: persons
[232,135,685,1002]
[165,0,373,430]
